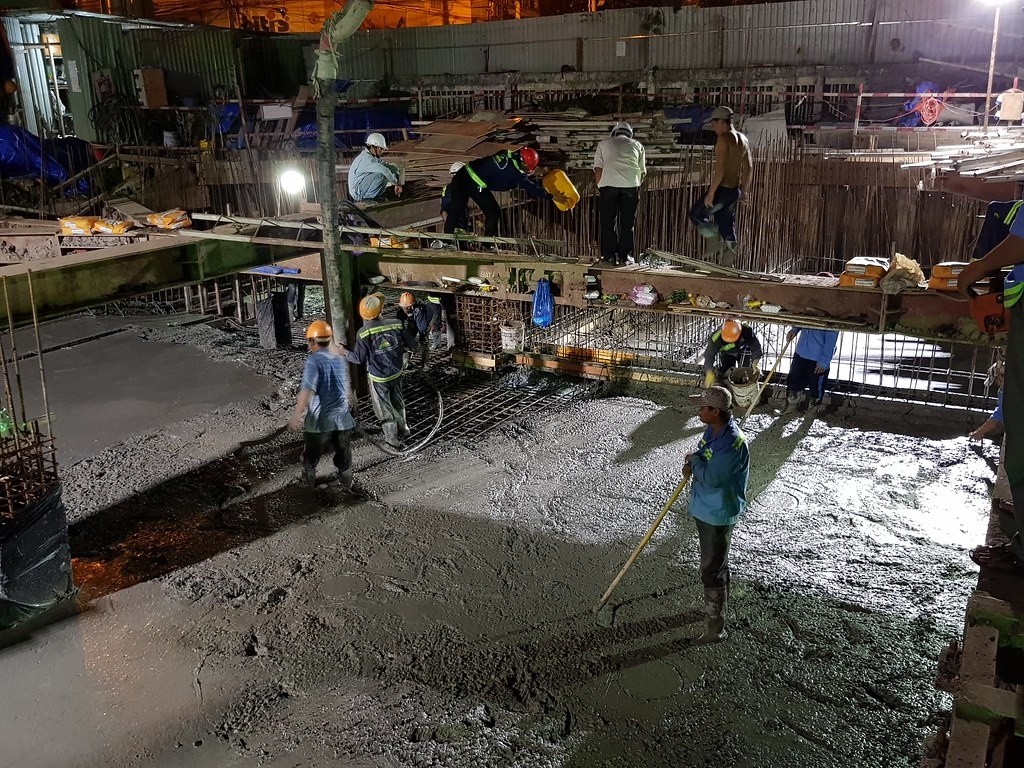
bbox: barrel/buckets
[728,367,759,408]
[164,131,178,147]
[542,169,580,212]
[498,320,525,354]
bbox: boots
[296,470,316,488]
[698,221,724,257]
[721,241,737,266]
[773,389,805,416]
[690,582,731,644]
[805,397,822,418]
[397,408,411,435]
[382,421,400,447]
[339,468,352,486]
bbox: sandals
[992,498,1015,519]
[969,544,1023,572]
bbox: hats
[704,106,733,123]
[983,362,1005,387]
[689,386,732,412]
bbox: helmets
[520,149,539,172]
[359,292,414,319]
[449,161,465,173]
[610,121,633,139]
[365,133,388,149]
[306,321,332,339]
[721,321,741,342]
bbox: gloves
[752,358,762,374]
[704,371,716,388]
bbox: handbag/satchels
[532,278,553,327]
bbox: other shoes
[481,246,502,252]
[358,196,390,206]
[601,253,628,266]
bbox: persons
[704,320,763,386]
[683,385,750,644]
[956,203,1024,574]
[970,363,1006,440]
[336,291,411,446]
[347,132,402,205]
[398,291,446,371]
[289,320,355,488]
[443,146,573,251]
[440,161,467,222]
[288,282,297,320]
[690,106,753,257]
[592,122,648,262]
[773,272,839,414]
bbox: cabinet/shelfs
[45,57,69,90]
